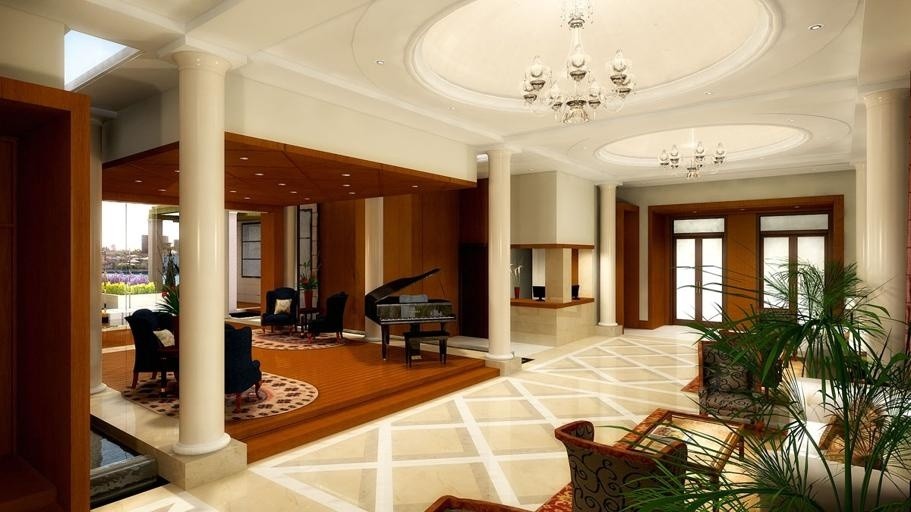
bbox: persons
[162,261,179,285]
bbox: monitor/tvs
[533,286,545,301]
[572,285,580,300]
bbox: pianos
[364,268,458,361]
